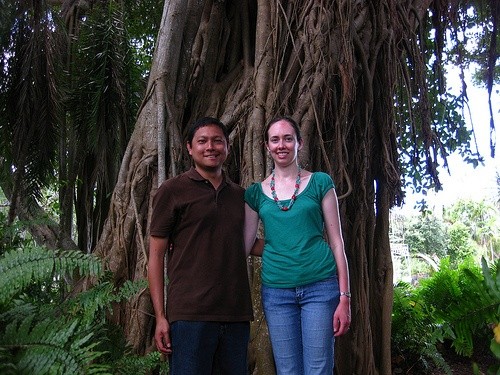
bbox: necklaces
[269,165,301,212]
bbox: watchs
[340,292,351,298]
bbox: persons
[148,116,267,375]
[245,117,352,375]
